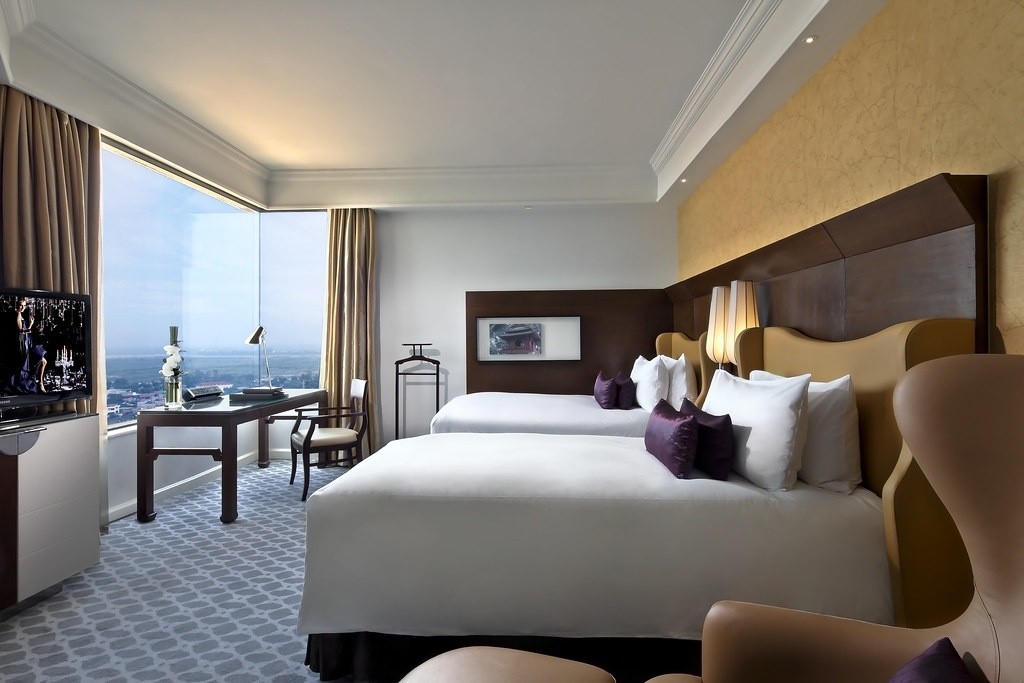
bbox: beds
[429,255,737,437]
[298,169,993,683]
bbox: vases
[165,376,182,408]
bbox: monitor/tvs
[0,288,91,424]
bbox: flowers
[158,326,187,403]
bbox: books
[229,386,289,401]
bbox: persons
[0,299,50,396]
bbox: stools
[397,646,617,683]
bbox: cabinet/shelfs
[0,412,101,623]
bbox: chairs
[288,379,368,501]
[644,354,1024,683]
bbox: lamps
[726,279,760,366]
[706,286,732,370]
[248,326,274,388]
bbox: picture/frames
[474,315,584,365]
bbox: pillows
[593,370,619,409]
[701,369,812,492]
[613,372,635,410]
[749,370,864,496]
[888,636,972,683]
[629,355,667,413]
[644,398,701,479]
[661,352,698,411]
[680,396,735,481]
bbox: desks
[136,388,329,524]
